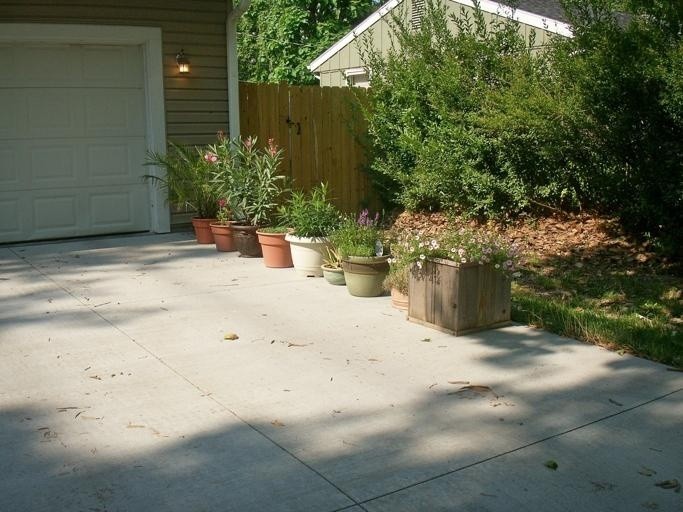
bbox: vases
[341,257,390,297]
[391,286,409,309]
[407,255,513,335]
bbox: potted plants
[141,129,344,286]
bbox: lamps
[176,48,190,74]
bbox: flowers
[380,205,531,297]
[323,208,393,255]
